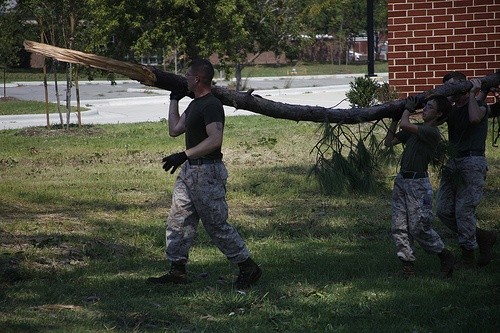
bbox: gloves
[162,151,189,174]
[404,95,419,113]
[170,83,188,101]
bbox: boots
[146,267,187,286]
[233,257,262,290]
[398,260,423,278]
[437,250,455,279]
[455,250,474,269]
[476,227,497,265]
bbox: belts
[189,158,222,166]
[400,171,428,179]
[448,151,485,158]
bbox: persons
[437,72,488,273]
[478,84,500,117]
[146,59,263,290]
[384,95,458,281]
